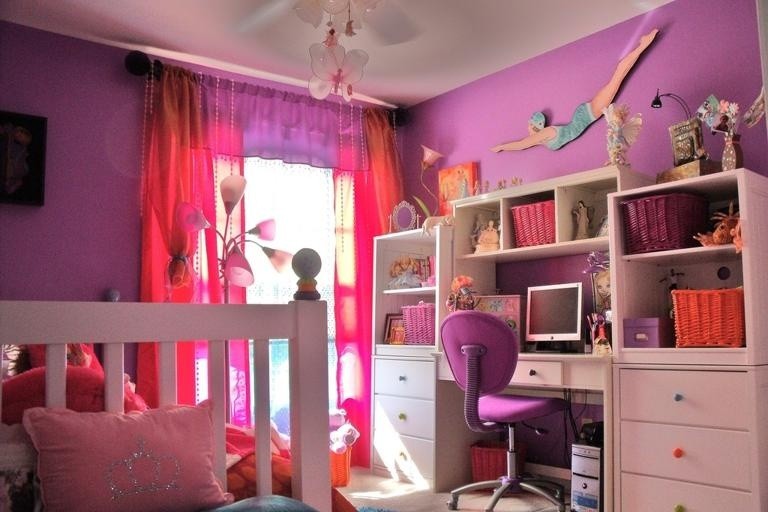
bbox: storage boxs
[471,293,528,354]
[622,315,674,348]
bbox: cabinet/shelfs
[613,365,766,511]
[368,223,502,494]
[430,163,657,512]
[606,167,768,366]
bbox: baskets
[669,286,746,349]
[467,438,529,495]
[401,301,437,347]
[618,193,711,255]
[509,198,555,249]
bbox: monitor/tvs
[524,283,582,353]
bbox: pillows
[0,419,43,512]
[21,395,235,512]
[26,342,105,377]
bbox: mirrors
[386,200,423,232]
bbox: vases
[721,133,743,172]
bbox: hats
[530,112,546,133]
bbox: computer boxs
[571,444,603,512]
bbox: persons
[488,28,660,154]
[387,256,421,289]
[659,268,685,293]
[572,201,589,240]
[593,271,611,313]
[715,115,728,132]
[703,101,716,119]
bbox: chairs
[440,310,571,512]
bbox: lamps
[291,1,380,103]
[176,174,294,424]
[651,87,692,121]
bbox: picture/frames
[383,312,406,345]
[711,112,731,134]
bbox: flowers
[711,99,740,138]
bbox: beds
[0,246,333,512]
